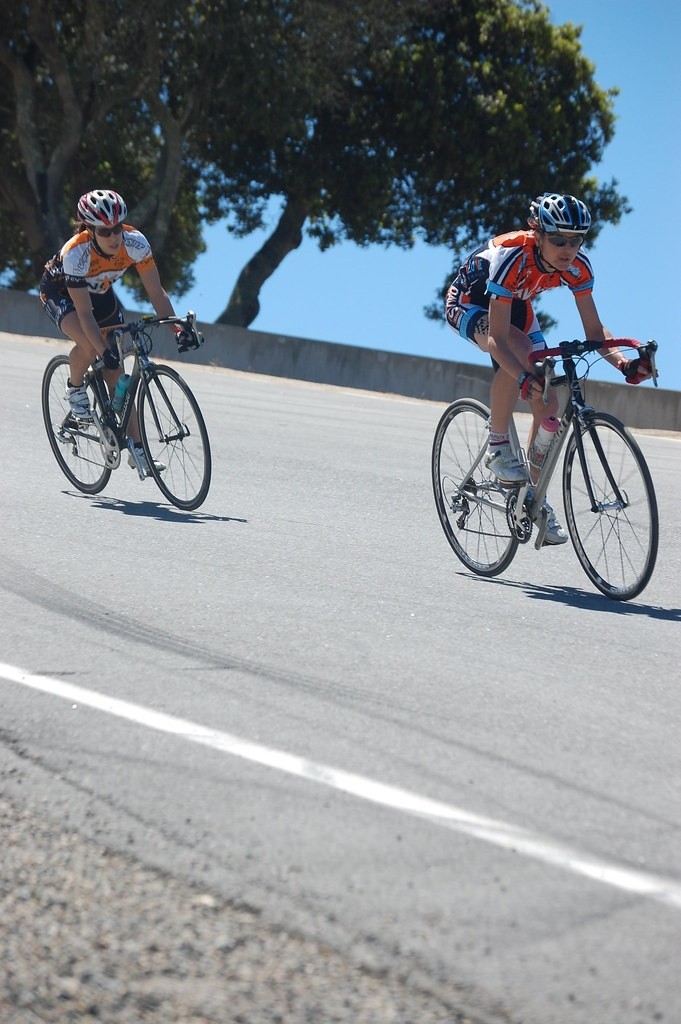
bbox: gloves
[102,349,120,369]
[174,328,196,348]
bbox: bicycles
[40,311,212,511]
[431,336,659,601]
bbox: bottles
[111,374,131,412]
[530,416,559,467]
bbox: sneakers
[63,377,92,418]
[526,497,568,545]
[127,447,166,473]
[483,444,529,485]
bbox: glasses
[89,223,124,237]
[539,232,583,248]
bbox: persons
[446,193,657,545]
[39,190,205,474]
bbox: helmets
[76,189,128,227]
[529,192,592,235]
